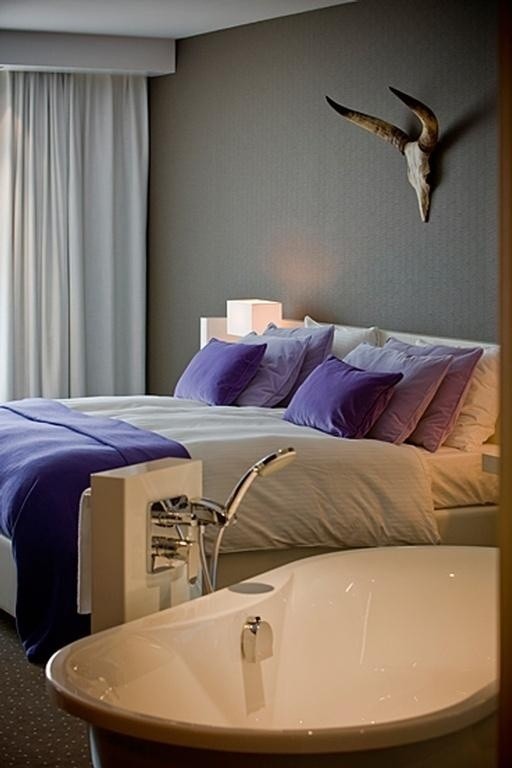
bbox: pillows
[384,337,484,453]
[339,341,456,446]
[261,323,338,409]
[411,339,502,453]
[302,317,384,363]
[174,338,269,405]
[235,330,313,407]
[283,355,406,439]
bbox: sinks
[45,546,500,752]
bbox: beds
[0,324,511,661]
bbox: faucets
[150,446,295,592]
